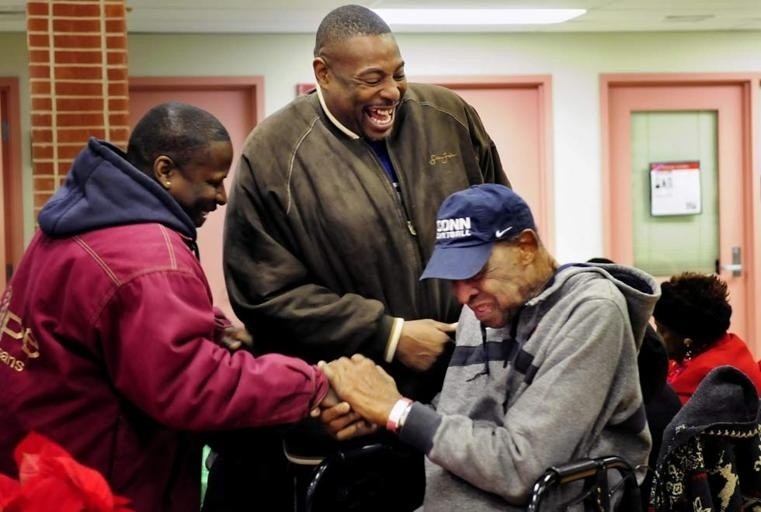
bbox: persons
[1,102,344,512]
[655,273,761,412]
[225,3,514,511]
[587,258,683,512]
[311,184,661,511]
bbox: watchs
[385,396,414,433]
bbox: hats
[419,183,535,280]
